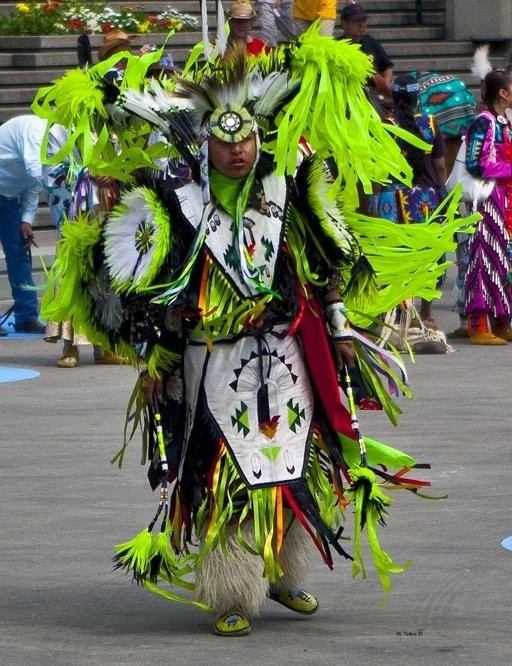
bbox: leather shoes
[0,327,9,337]
[14,318,47,334]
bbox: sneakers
[212,606,253,637]
[56,345,79,369]
[93,345,132,365]
[266,587,320,616]
[446,327,512,346]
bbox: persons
[336,3,512,353]
[0,114,132,367]
[99,1,336,69]
[32,34,483,639]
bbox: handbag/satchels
[445,134,498,205]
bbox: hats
[340,3,369,22]
[97,29,140,59]
[147,53,182,72]
[224,0,258,21]
